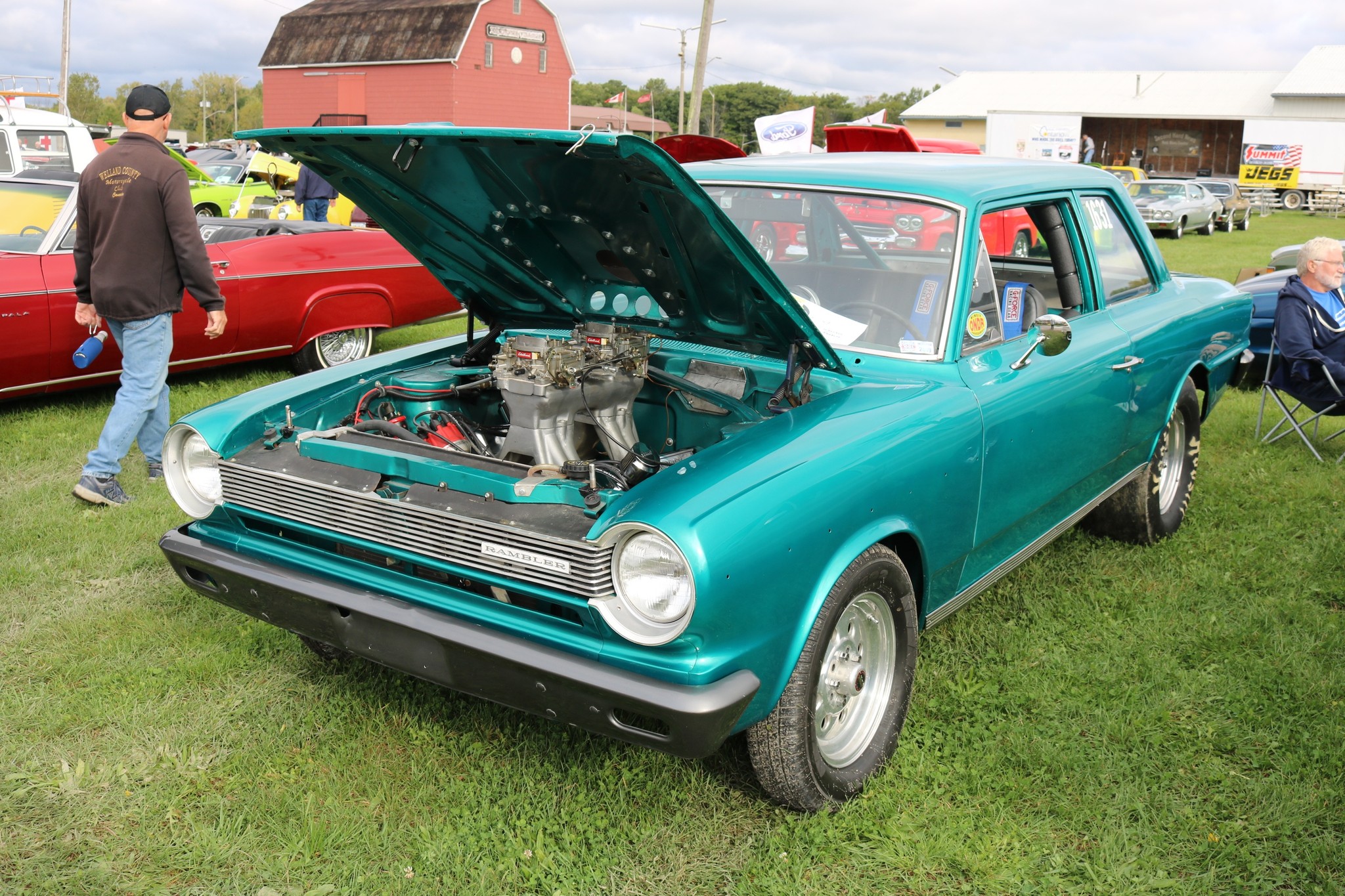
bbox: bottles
[75,331,110,368]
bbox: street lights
[203,111,225,148]
[234,76,250,131]
[641,19,727,135]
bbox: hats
[126,84,171,120]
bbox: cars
[652,125,1252,262]
[1235,240,1345,390]
[159,123,1212,819]
[1,76,470,399]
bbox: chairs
[1254,315,1345,464]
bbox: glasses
[1314,259,1345,267]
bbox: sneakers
[72,476,141,507]
[148,461,165,481]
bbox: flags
[638,93,651,102]
[605,91,624,104]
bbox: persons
[1081,133,1095,164]
[18,138,26,151]
[35,142,46,151]
[70,85,227,506]
[293,164,340,223]
[225,138,258,160]
[1276,236,1345,415]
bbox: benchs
[198,223,258,244]
[767,261,1047,347]
